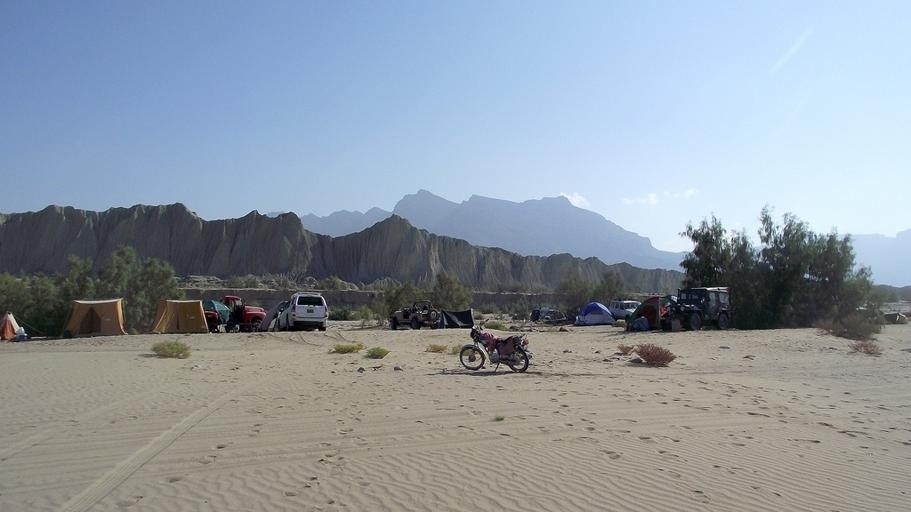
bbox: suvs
[671,286,733,332]
[609,300,644,319]
[389,298,441,330]
[277,291,330,330]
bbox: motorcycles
[459,318,531,374]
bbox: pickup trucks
[204,294,267,334]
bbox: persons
[632,313,649,331]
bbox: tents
[626,294,675,332]
[574,300,615,326]
[60,297,131,337]
[148,297,211,334]
[203,298,231,325]
